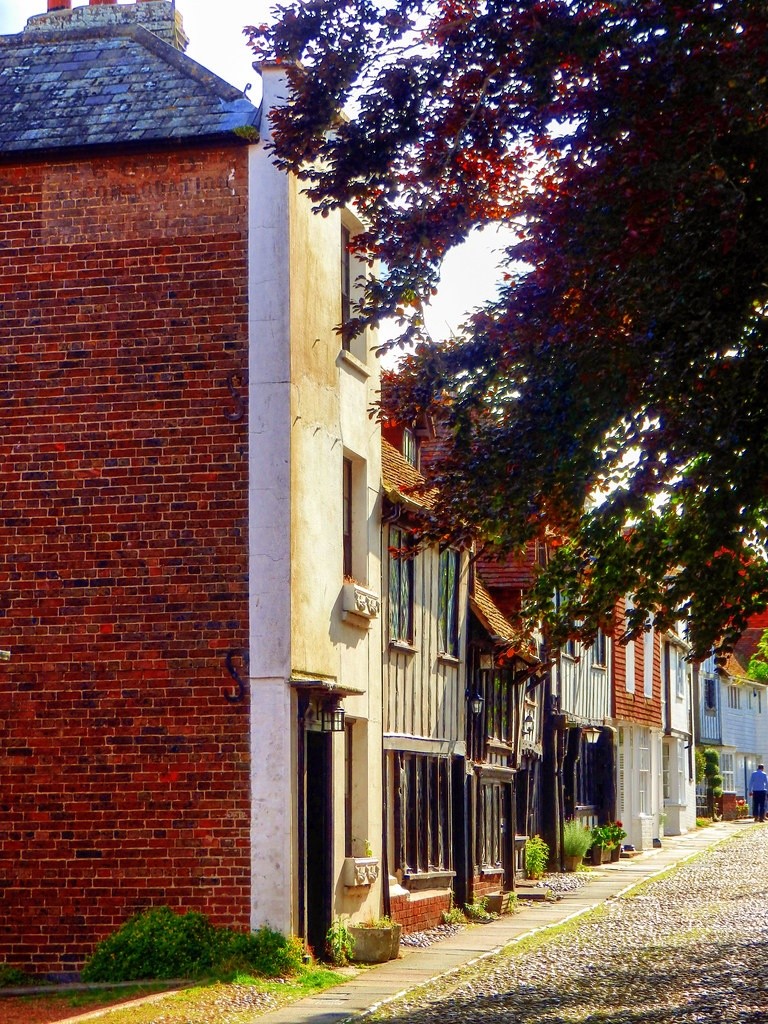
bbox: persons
[748,764,768,822]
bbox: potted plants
[601,827,612,862]
[523,836,551,880]
[358,914,402,959]
[590,825,606,865]
[563,819,595,872]
[347,919,393,964]
[610,821,627,862]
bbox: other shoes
[754,811,767,824]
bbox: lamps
[466,685,485,720]
[314,695,346,733]
[521,715,535,736]
